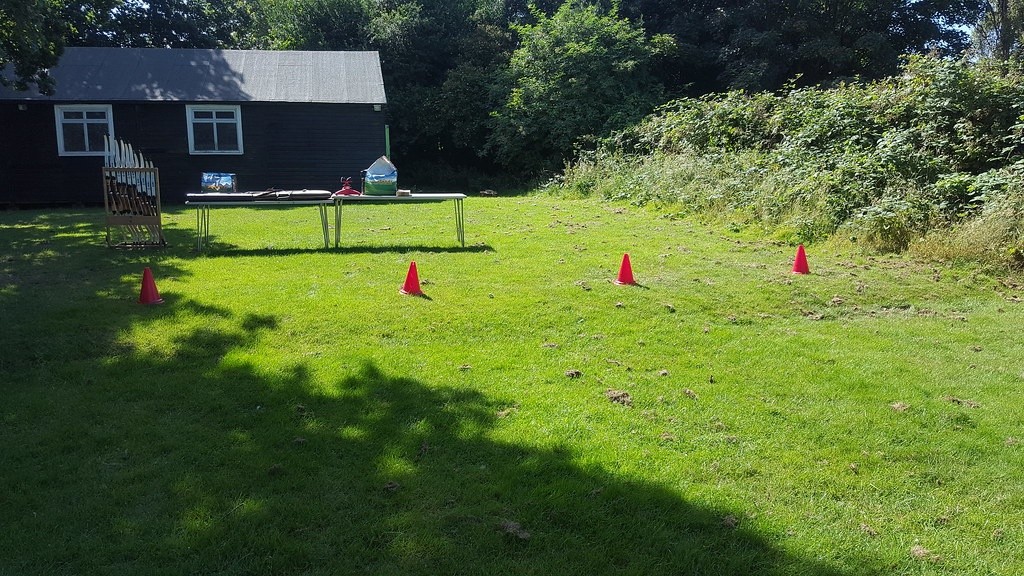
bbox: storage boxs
[201,172,236,193]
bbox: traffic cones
[399,261,424,296]
[614,253,637,285]
[791,244,812,275]
[134,265,162,304]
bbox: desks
[185,200,335,249]
[335,193,467,250]
